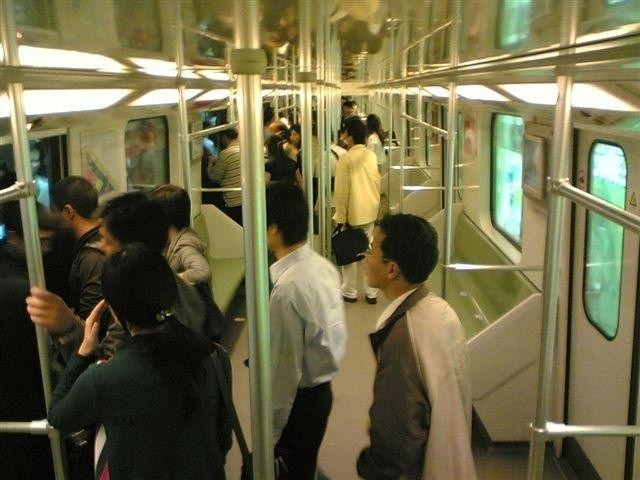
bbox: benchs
[192,202,245,317]
[379,155,437,217]
[417,204,552,447]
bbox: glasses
[366,248,389,262]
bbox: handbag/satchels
[194,280,224,338]
[331,228,369,266]
[240,452,290,480]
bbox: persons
[240,179,346,480]
[357,214,477,479]
[1,142,235,479]
[198,0,389,81]
[203,95,388,304]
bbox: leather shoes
[365,293,378,305]
[342,295,358,303]
